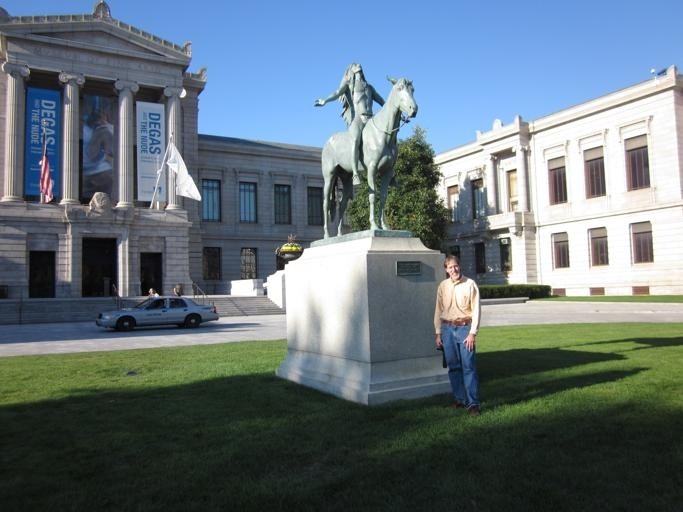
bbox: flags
[39,154,53,203]
[165,144,201,202]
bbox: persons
[169,300,176,307]
[173,285,182,296]
[81,96,115,175]
[433,255,482,417]
[157,300,165,308]
[313,63,408,187]
[148,289,159,297]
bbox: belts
[442,319,472,326]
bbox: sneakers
[467,404,482,417]
[446,400,466,409]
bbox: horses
[321,74,419,240]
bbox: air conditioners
[500,238,509,244]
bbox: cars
[96,296,219,331]
